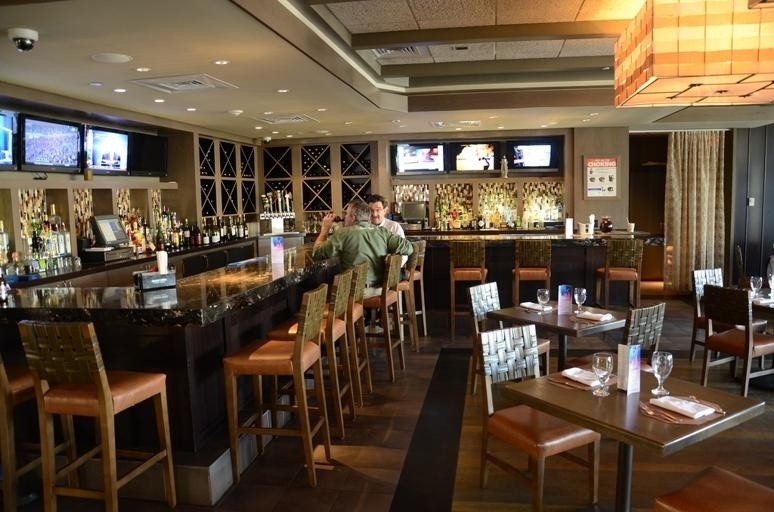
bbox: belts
[365,283,384,288]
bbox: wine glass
[592,352,614,396]
[750,276,763,300]
[574,288,587,314]
[537,289,550,315]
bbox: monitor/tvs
[402,201,426,221]
[448,141,500,170]
[83,124,130,176]
[506,140,559,169]
[0,109,17,171]
[392,143,446,173]
[19,113,81,174]
[131,132,168,176]
[92,214,129,245]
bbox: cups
[627,223,635,232]
[577,223,586,234]
[651,351,674,394]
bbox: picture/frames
[581,153,621,199]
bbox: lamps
[613,0,774,108]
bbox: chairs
[255,240,427,442]
[617,302,666,372]
[700,283,773,399]
[475,324,602,511]
[223,283,332,489]
[595,239,644,312]
[0,356,78,511]
[451,239,488,322]
[466,282,551,396]
[689,268,768,364]
[513,238,552,304]
[653,464,773,511]
[17,318,177,511]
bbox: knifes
[547,376,590,391]
[649,409,678,421]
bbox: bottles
[501,156,508,178]
[35,288,76,308]
[333,215,345,222]
[84,163,94,181]
[434,195,564,229]
[0,203,96,275]
[600,216,613,232]
[116,205,250,254]
[1,280,8,303]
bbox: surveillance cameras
[8,28,39,53]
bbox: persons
[312,202,414,300]
[366,195,408,283]
[388,202,401,221]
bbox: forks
[642,404,678,424]
